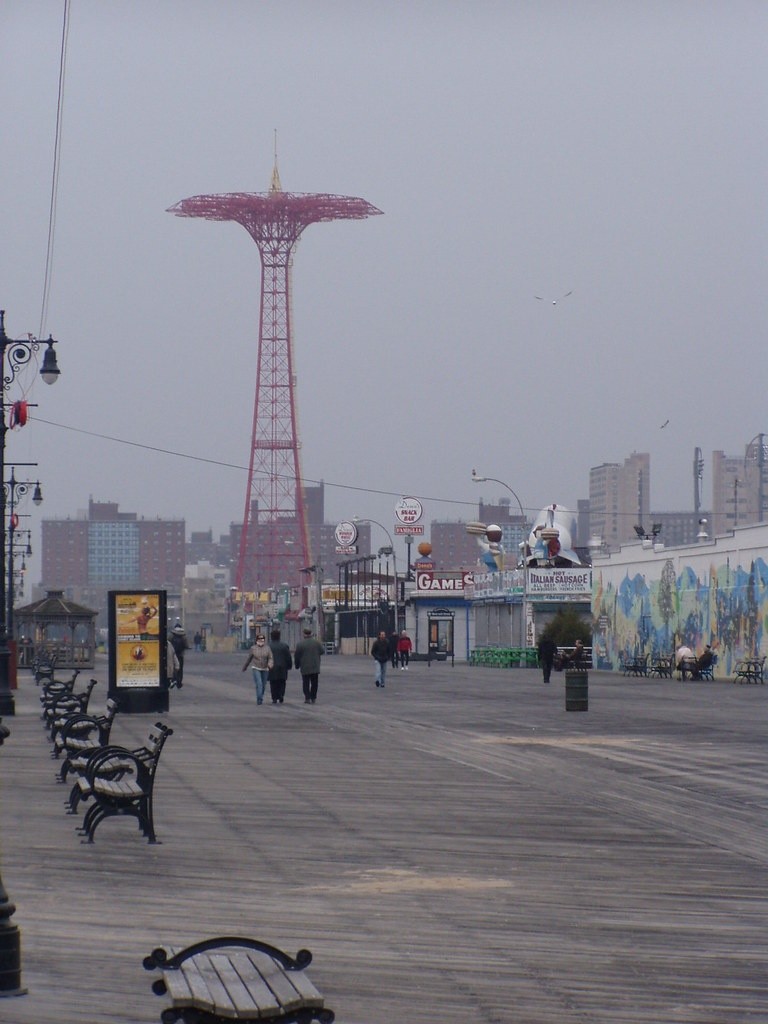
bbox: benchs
[623,653,717,682]
[566,651,588,672]
[40,669,174,844]
[732,656,766,685]
[29,646,58,685]
[142,936,335,1024]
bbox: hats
[302,627,311,635]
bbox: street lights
[0,307,62,747]
[470,468,528,669]
[352,515,399,663]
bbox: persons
[556,640,584,672]
[371,631,391,688]
[168,624,186,689]
[397,630,413,671]
[538,634,558,683]
[268,630,292,703]
[390,630,400,668]
[119,605,158,641]
[166,640,179,689]
[294,627,325,704]
[193,632,202,651]
[675,644,713,681]
[242,634,274,705]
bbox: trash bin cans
[565,669,589,711]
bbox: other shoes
[258,698,262,704]
[401,665,408,671]
[304,698,315,703]
[272,698,284,704]
[170,680,177,689]
[376,681,380,687]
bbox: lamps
[634,526,645,535]
[652,524,661,536]
